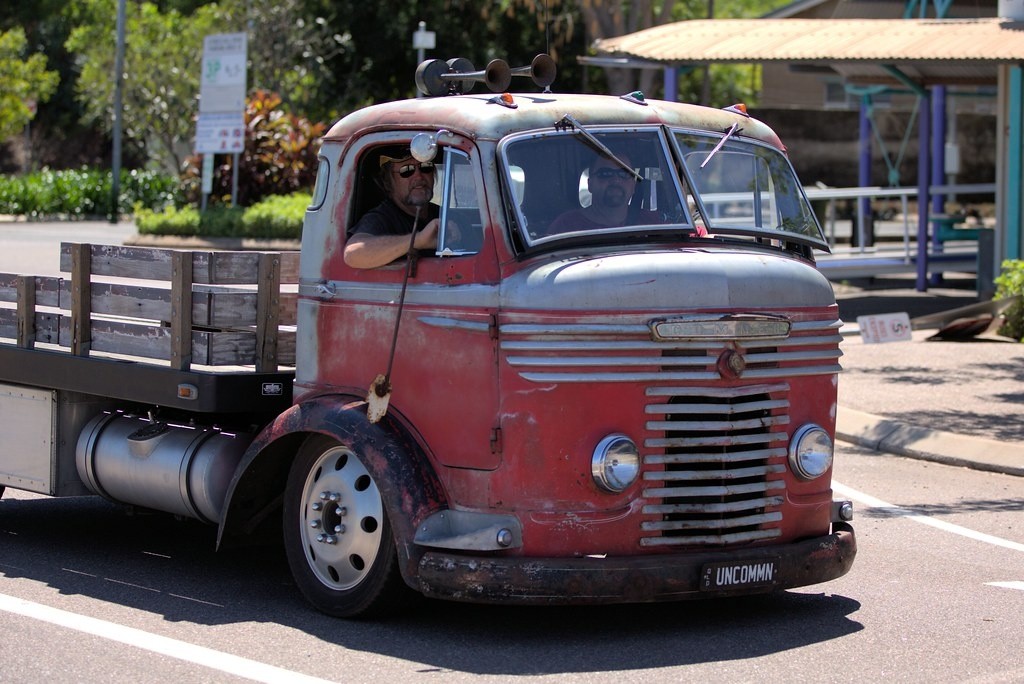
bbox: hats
[380,144,412,168]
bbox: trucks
[0,53,858,625]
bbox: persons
[546,142,708,237]
[343,151,479,268]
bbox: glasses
[391,160,434,178]
[591,167,634,180]
